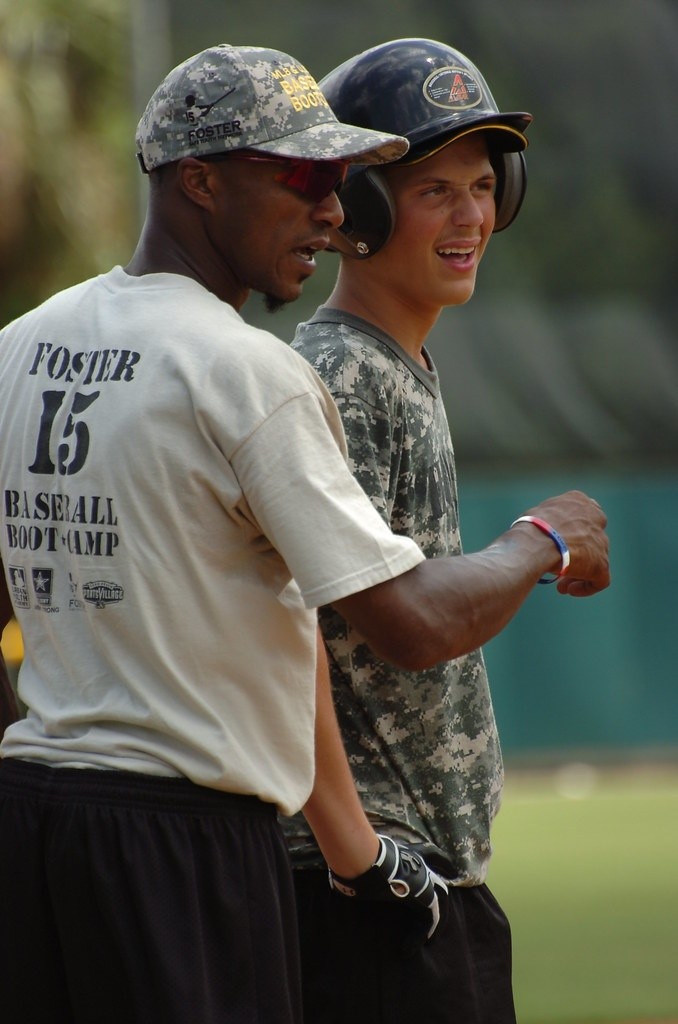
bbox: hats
[135,43,411,171]
[381,123,530,167]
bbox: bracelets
[511,515,571,584]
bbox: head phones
[326,147,527,260]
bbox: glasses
[202,153,350,203]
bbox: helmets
[317,38,528,259]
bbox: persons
[0,44,612,1024]
[278,37,534,1024]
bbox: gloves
[328,835,458,941]
